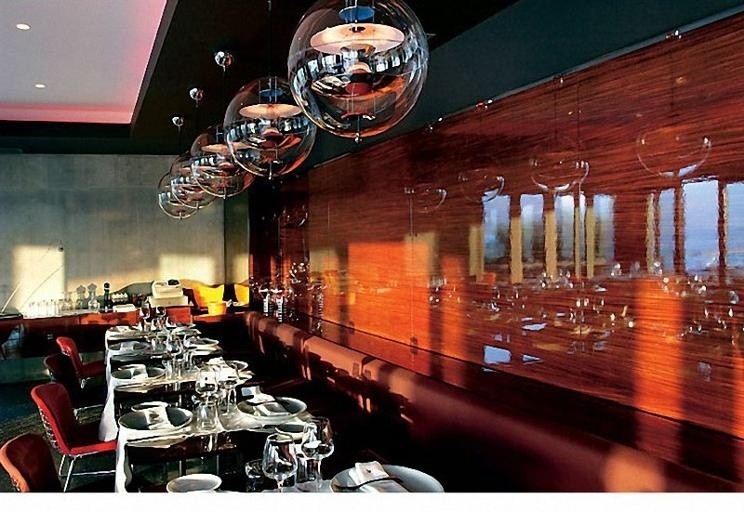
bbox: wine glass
[138,301,235,437]
[246,413,335,492]
[395,258,741,341]
[59,289,98,313]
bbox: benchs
[362,358,744,491]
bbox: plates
[328,461,445,492]
[166,473,221,492]
[178,322,310,420]
[106,326,193,432]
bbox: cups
[22,299,60,320]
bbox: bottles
[103,280,110,308]
